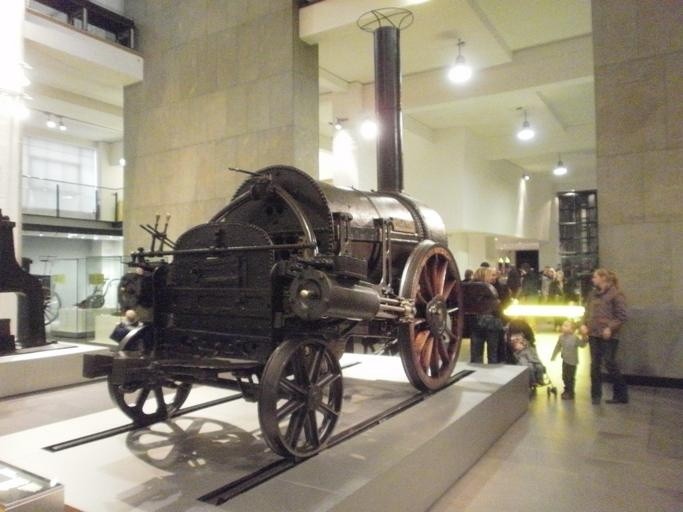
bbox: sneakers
[591,395,627,405]
[561,392,574,399]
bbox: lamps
[446,38,569,178]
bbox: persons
[550,319,587,400]
[506,328,547,386]
[467,266,498,363]
[487,266,512,363]
[576,267,630,405]
[460,260,596,308]
